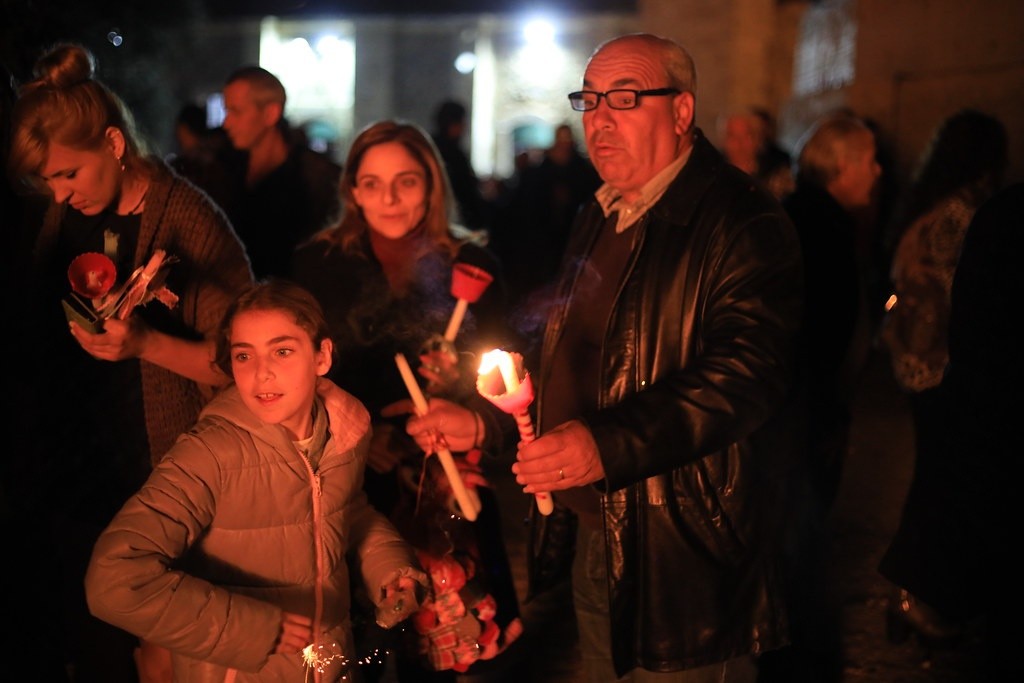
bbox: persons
[380,33,806,683]
[746,109,1024,682]
[0,42,604,683]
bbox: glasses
[568,87,681,112]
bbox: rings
[393,599,403,613]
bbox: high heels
[888,581,961,650]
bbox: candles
[394,352,475,522]
[86,270,102,309]
[121,248,166,319]
[496,350,555,515]
[104,225,118,317]
[439,298,467,352]
